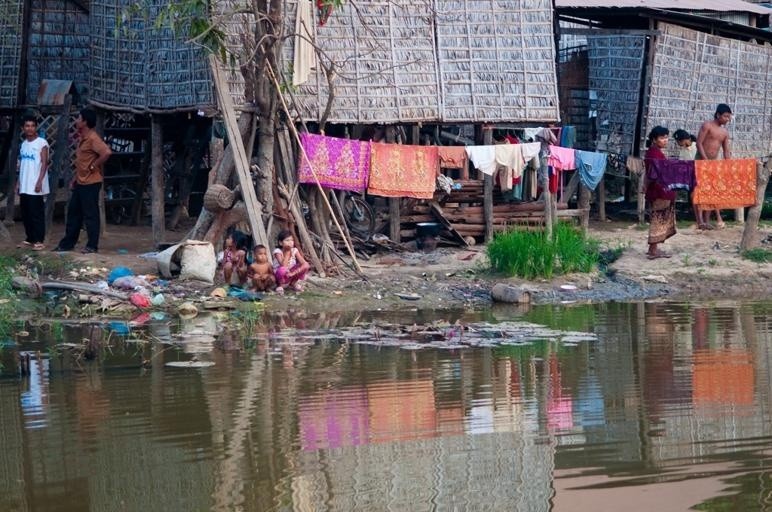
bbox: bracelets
[89,164,96,172]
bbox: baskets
[203,183,235,213]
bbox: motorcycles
[301,186,376,235]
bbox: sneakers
[51,246,72,252]
[81,247,96,254]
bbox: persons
[692,103,733,229]
[272,229,311,294]
[219,233,248,289]
[14,117,50,253]
[54,106,114,254]
[672,128,725,229]
[641,125,678,260]
[246,244,274,293]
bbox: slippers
[289,284,305,292]
[17,240,46,251]
[275,286,284,294]
[646,249,671,259]
[698,222,726,230]
